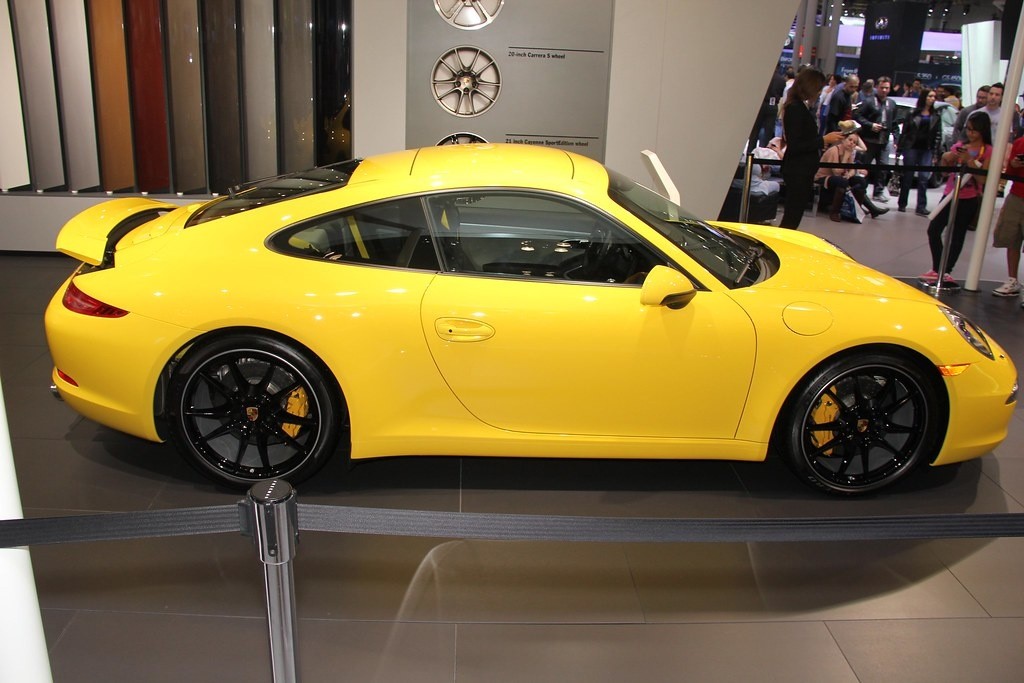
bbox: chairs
[438,203,473,272]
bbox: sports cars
[43,140,1019,499]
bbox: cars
[923,81,962,97]
[852,98,960,184]
[784,30,796,47]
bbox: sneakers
[919,268,939,277]
[993,278,1020,296]
[944,272,955,282]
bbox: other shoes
[916,208,931,214]
[873,195,888,202]
[898,204,906,212]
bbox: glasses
[963,127,978,134]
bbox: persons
[747,67,1024,308]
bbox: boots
[829,187,845,222]
[859,195,889,218]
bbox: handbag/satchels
[888,160,900,197]
